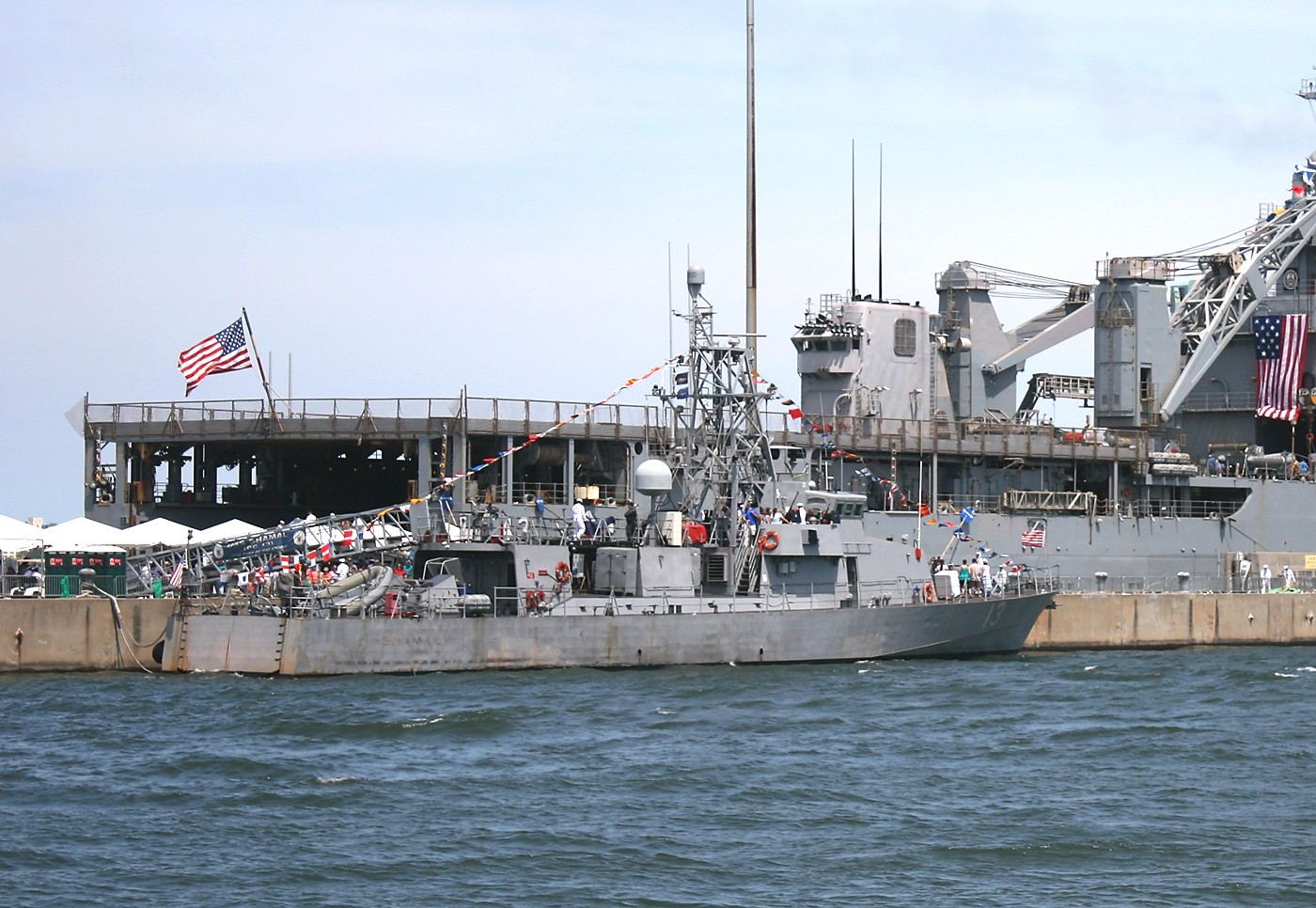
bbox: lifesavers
[556,561,570,582]
[922,583,934,602]
[763,530,779,550]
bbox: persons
[1282,565,1297,588]
[1260,564,1272,594]
[624,502,638,542]
[722,499,840,548]
[570,497,587,542]
[930,548,1011,599]
[1208,452,1234,478]
[1292,458,1310,479]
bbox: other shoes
[978,595,981,597]
[576,536,580,539]
[970,594,974,597]
[573,536,577,541]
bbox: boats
[160,228,1061,680]
[80,0,1316,592]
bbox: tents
[0,511,476,570]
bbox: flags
[1022,530,1047,549]
[178,316,255,399]
[1250,313,1308,421]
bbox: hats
[797,503,803,506]
[1263,564,1268,568]
[1284,565,1288,569]
[577,498,583,502]
